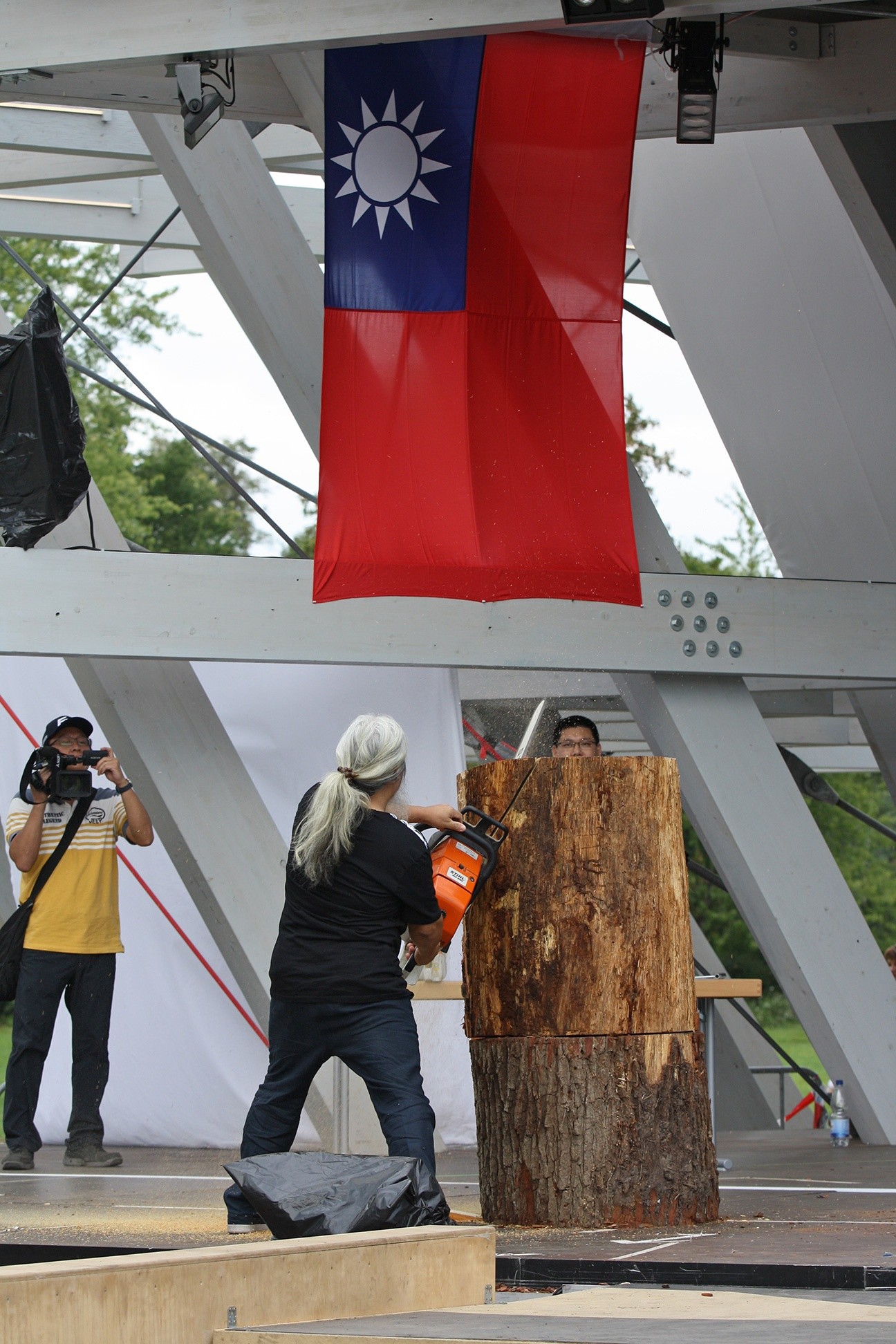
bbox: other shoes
[227,1212,269,1233]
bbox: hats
[42,715,94,744]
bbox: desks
[332,979,762,1170]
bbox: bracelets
[115,778,133,794]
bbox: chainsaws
[398,698,565,979]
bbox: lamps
[167,54,225,150]
[561,0,665,25]
[669,13,729,145]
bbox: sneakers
[1,1145,35,1170]
[63,1141,123,1168]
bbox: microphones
[40,745,58,759]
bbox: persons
[551,715,602,756]
[221,712,466,1233]
[1,714,157,1170]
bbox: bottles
[831,1079,850,1148]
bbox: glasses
[554,740,599,750]
[47,734,92,748]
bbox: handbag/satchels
[0,898,36,1002]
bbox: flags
[309,21,647,611]
